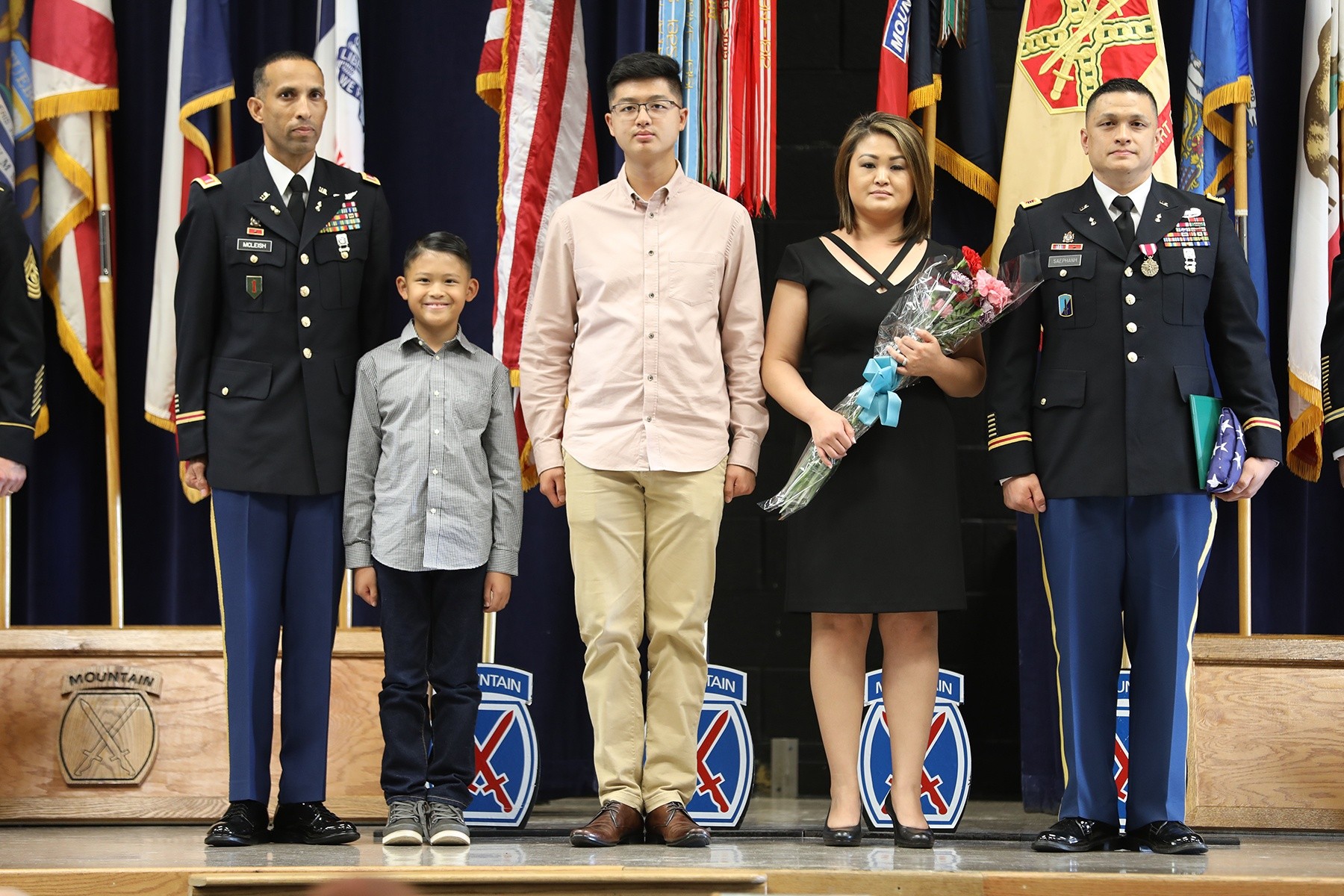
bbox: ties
[286,175,308,236]
[1112,196,1136,254]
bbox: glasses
[610,100,681,121]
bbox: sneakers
[422,800,471,846]
[381,801,424,846]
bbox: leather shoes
[570,800,645,847]
[1129,821,1209,853]
[272,801,360,844]
[645,801,711,847]
[204,800,269,845]
[1032,817,1119,853]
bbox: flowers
[774,245,1015,520]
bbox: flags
[0,1,120,407]
[981,0,1178,354]
[312,0,366,174]
[877,0,1000,211]
[1178,0,1270,398]
[1287,0,1344,482]
[475,0,600,492]
[144,0,236,435]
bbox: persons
[517,51,769,847]
[0,181,52,497]
[1320,253,1344,488]
[976,77,1281,855]
[759,111,987,850]
[342,231,524,845]
[172,52,399,846]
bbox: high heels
[885,791,934,848]
[821,792,863,846]
